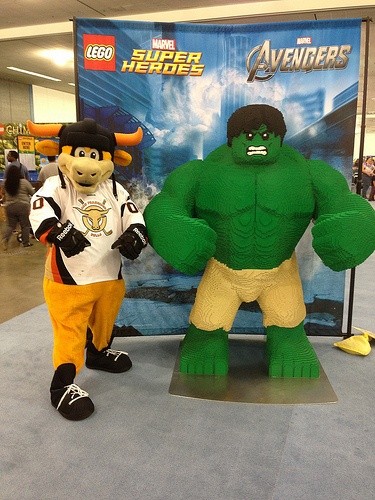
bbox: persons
[38,156,58,189]
[352,156,375,201]
[6,151,35,242]
[143,104,375,379]
[0,165,35,249]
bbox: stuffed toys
[27,118,147,421]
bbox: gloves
[52,220,91,259]
[111,223,150,261]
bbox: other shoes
[23,243,34,249]
[2,238,9,250]
